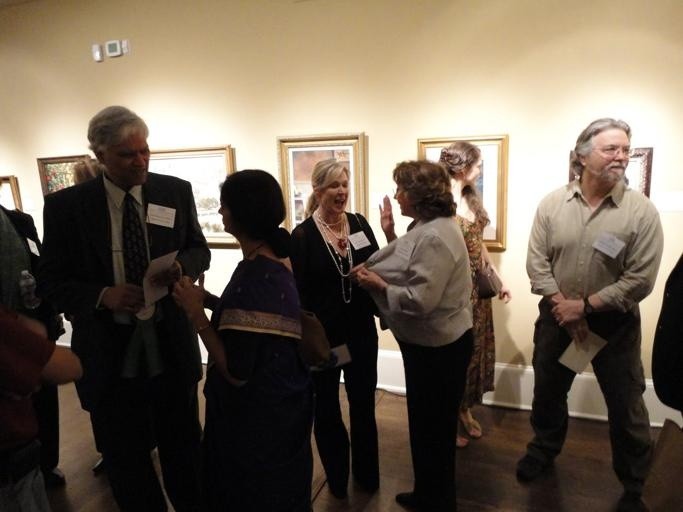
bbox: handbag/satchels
[300,311,332,367]
[640,419,683,512]
[477,262,502,298]
[10,439,41,483]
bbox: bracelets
[193,324,211,333]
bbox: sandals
[459,409,482,438]
[457,436,469,448]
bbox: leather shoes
[93,457,105,472]
[49,468,65,487]
[396,493,457,512]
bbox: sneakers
[615,490,645,512]
[516,454,554,481]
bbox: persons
[0,303,84,512]
[171,170,315,512]
[438,141,513,449]
[289,158,388,501]
[356,160,473,512]
[0,205,65,489]
[33,105,212,512]
[74,158,157,476]
[516,118,665,512]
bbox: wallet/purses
[310,344,351,372]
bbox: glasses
[589,147,635,158]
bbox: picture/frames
[148,144,242,248]
[417,136,509,253]
[37,154,92,196]
[277,132,366,233]
[0,175,23,212]
[569,147,655,199]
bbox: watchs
[584,298,593,316]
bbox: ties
[122,192,161,327]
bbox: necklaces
[244,243,265,260]
[313,211,353,304]
